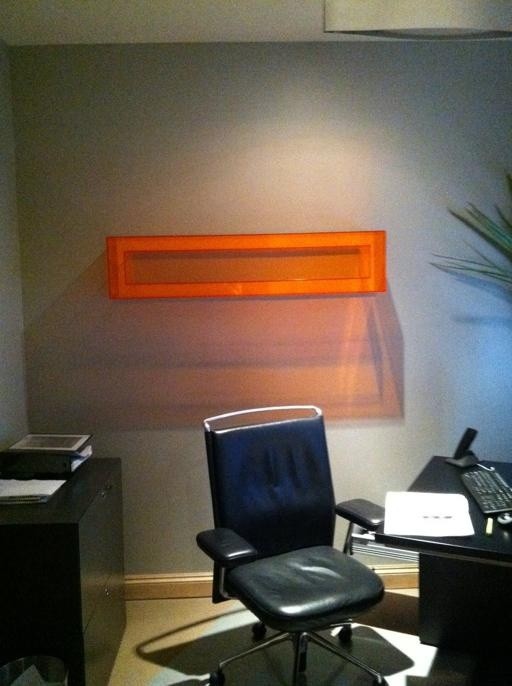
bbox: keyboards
[461,470,512,514]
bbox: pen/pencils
[486,516,494,536]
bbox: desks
[374,456,511,658]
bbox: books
[0,479,67,505]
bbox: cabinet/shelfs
[0,457,127,686]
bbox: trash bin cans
[0,656,70,686]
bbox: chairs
[192,405,387,686]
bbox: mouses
[496,512,512,526]
